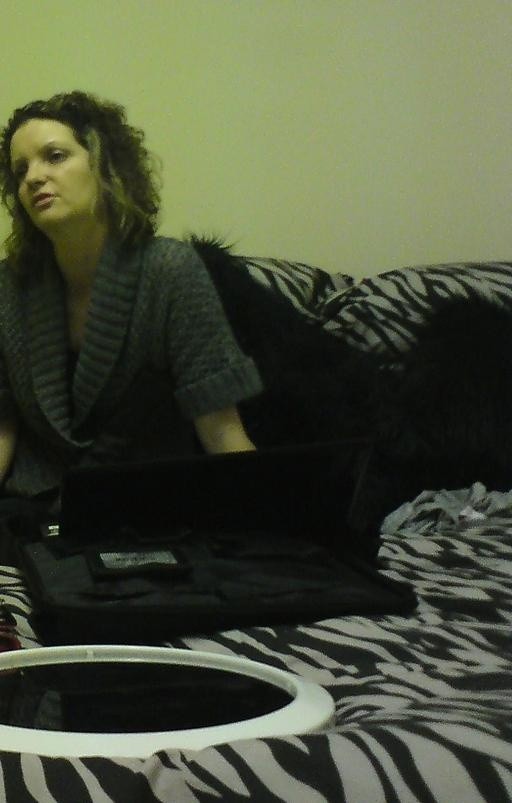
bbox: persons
[0,90,263,568]
